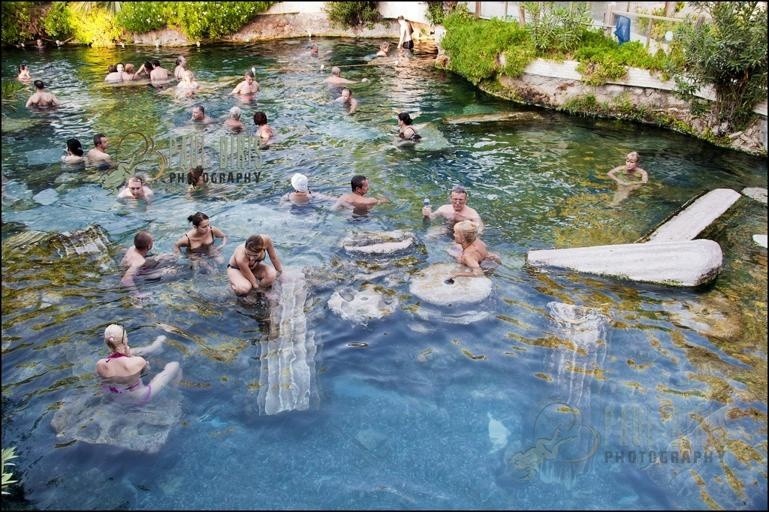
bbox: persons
[25,80,60,113]
[306,43,321,56]
[424,186,483,240]
[86,133,113,168]
[172,211,227,271]
[252,113,273,145]
[279,172,318,207]
[377,41,391,57]
[120,231,174,299]
[116,176,155,201]
[226,234,284,296]
[95,323,183,407]
[61,139,87,171]
[223,106,244,131]
[191,105,212,124]
[184,165,218,202]
[17,63,31,83]
[229,72,261,103]
[34,38,47,49]
[330,175,391,214]
[397,16,414,50]
[319,88,359,116]
[607,151,648,206]
[104,54,213,98]
[323,66,358,84]
[448,221,497,281]
[398,113,420,144]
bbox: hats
[291,173,309,193]
